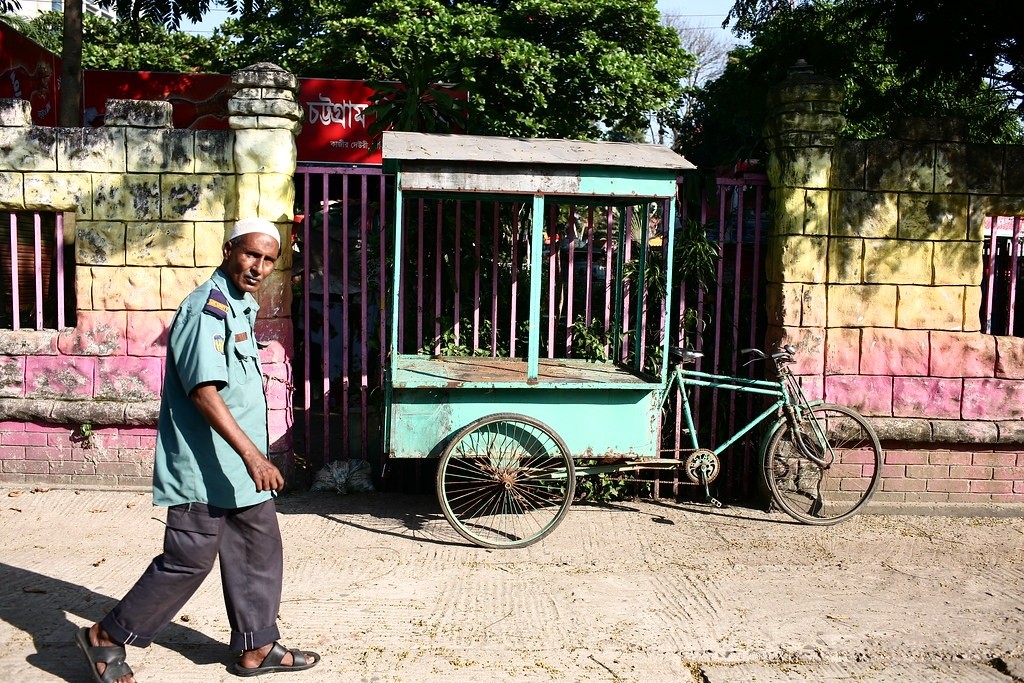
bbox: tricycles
[378,127,883,549]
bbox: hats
[228,217,281,249]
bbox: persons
[72,218,325,683]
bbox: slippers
[231,642,321,676]
[75,626,139,683]
